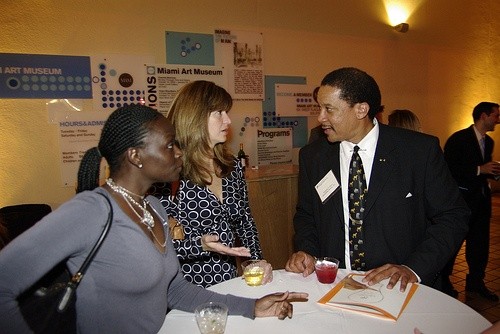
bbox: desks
[156,269,493,334]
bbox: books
[318,274,417,321]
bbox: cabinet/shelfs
[243,164,299,270]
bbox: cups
[313,256,340,284]
[241,260,264,287]
[193,301,228,334]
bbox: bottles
[238,144,246,172]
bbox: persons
[285,67,458,290]
[387,110,420,130]
[147,80,273,289]
[443,102,500,303]
[0,104,309,334]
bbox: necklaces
[106,176,169,247]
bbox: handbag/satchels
[15,191,113,331]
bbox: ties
[480,137,486,158]
[348,145,368,270]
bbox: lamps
[394,23,409,32]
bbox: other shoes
[466,285,498,301]
